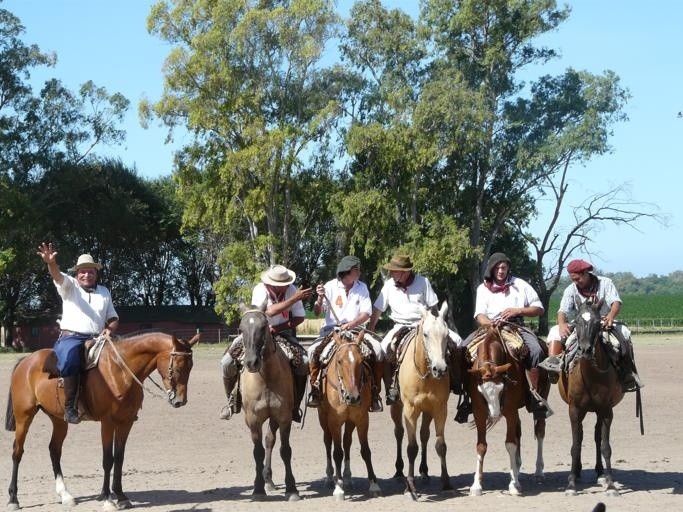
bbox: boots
[372,362,382,409]
[308,363,322,401]
[527,368,545,412]
[64,366,80,423]
[292,375,306,423]
[219,377,241,419]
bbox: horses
[317,328,384,501]
[467,328,551,498]
[238,294,301,502]
[5,327,203,511]
[390,300,458,500]
[558,294,627,495]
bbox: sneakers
[536,355,562,372]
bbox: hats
[484,252,511,281]
[567,260,592,272]
[260,265,295,286]
[68,255,102,272]
[336,256,360,281]
[382,255,415,272]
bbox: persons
[367,255,462,405]
[457,252,546,409]
[546,260,638,393]
[306,256,386,413]
[221,264,314,422]
[36,243,120,423]
